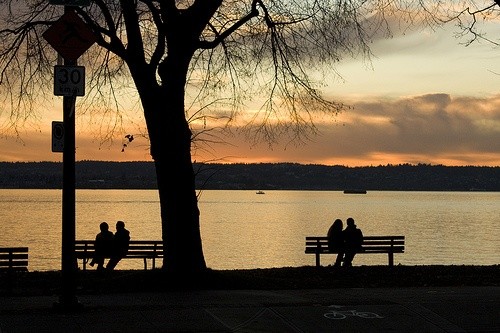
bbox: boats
[256,190,264,194]
[344,186,367,194]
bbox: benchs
[75,240,163,270]
[305,236,405,268]
[0,248,29,272]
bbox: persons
[326,217,365,267]
[90,221,130,270]
